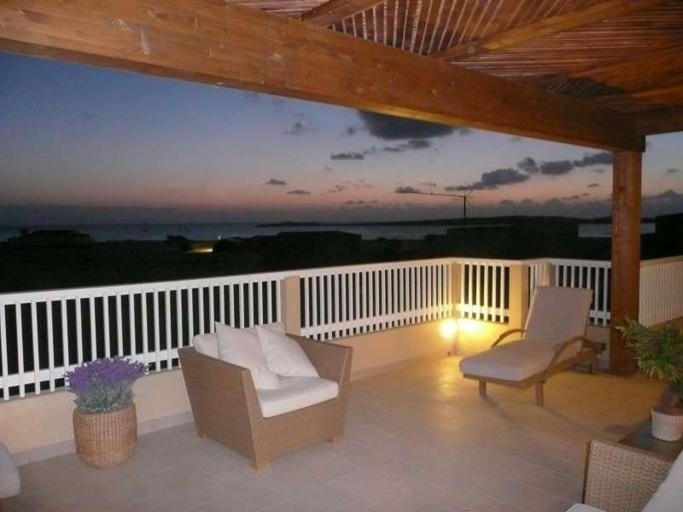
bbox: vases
[73,403,138,468]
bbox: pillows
[193,321,319,390]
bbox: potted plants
[615,314,683,442]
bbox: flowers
[62,354,152,414]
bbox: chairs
[460,285,607,407]
[0,441,21,512]
[563,438,683,512]
[178,321,353,470]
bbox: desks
[616,419,683,464]
